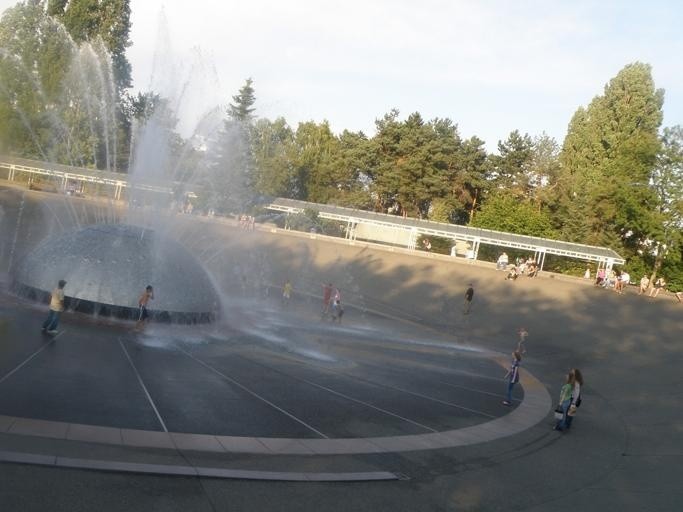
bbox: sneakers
[502,400,513,406]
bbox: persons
[649,276,666,298]
[178,200,255,231]
[516,325,526,356]
[421,237,431,250]
[595,266,630,295]
[136,285,154,331]
[565,367,582,430]
[308,224,316,239]
[335,300,343,323]
[321,282,331,315]
[282,279,292,305]
[40,279,66,334]
[494,251,539,282]
[637,274,649,295]
[551,373,571,431]
[500,351,519,406]
[463,282,473,315]
[332,288,341,310]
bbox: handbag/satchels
[567,404,577,417]
[575,394,582,407]
[555,409,566,420]
